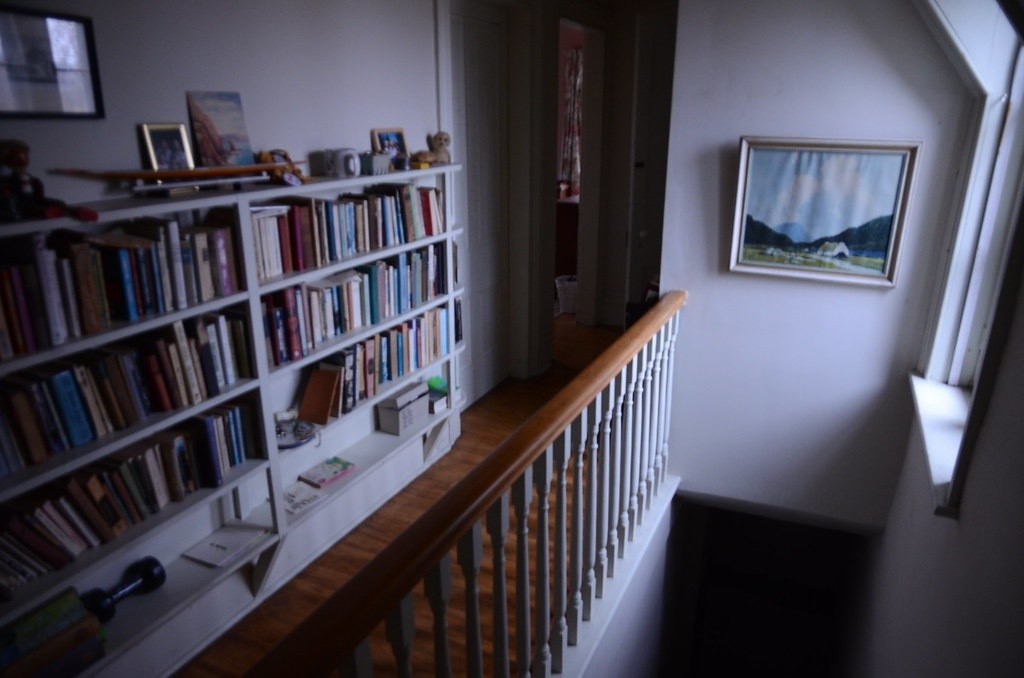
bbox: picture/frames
[141,121,199,196]
[370,128,410,172]
[728,136,924,289]
[0,6,106,122]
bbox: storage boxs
[377,382,431,436]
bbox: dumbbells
[82,554,168,623]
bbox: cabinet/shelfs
[0,163,468,678]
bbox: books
[0,585,109,678]
[252,180,459,426]
[266,480,329,515]
[0,198,249,600]
[181,517,270,573]
[298,456,357,489]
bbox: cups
[325,149,360,177]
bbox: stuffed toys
[0,137,98,226]
